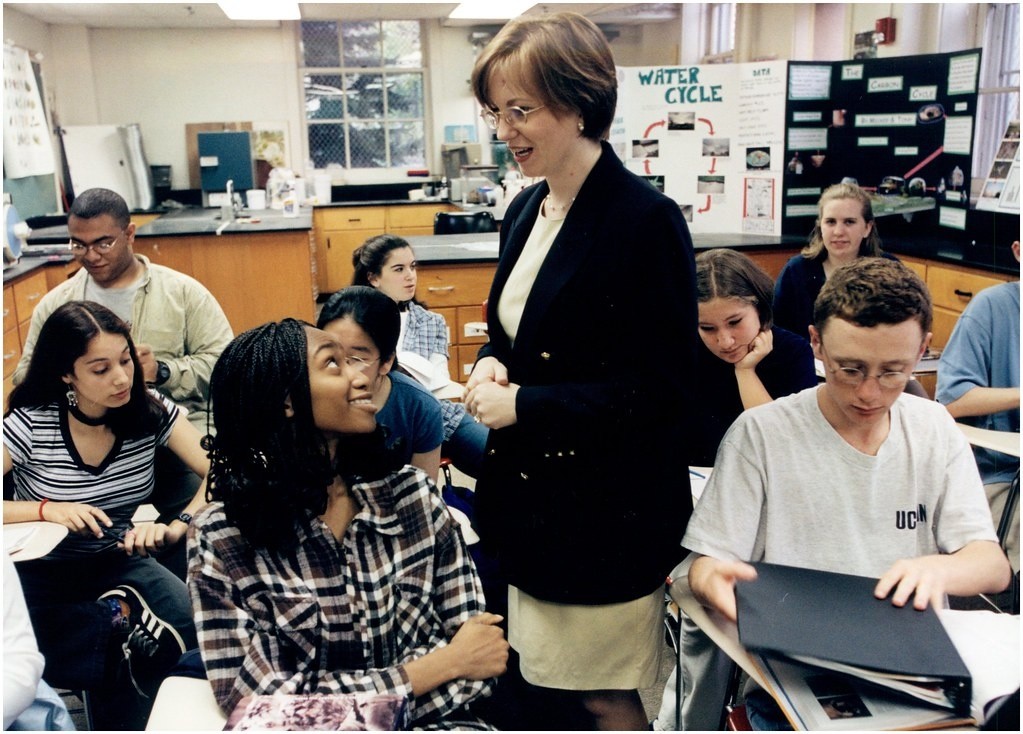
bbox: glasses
[820,342,915,390]
[479,104,546,130]
[345,356,381,372]
[69,231,124,256]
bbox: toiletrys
[282,183,299,217]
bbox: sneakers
[97,584,186,699]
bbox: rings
[126,531,135,536]
[473,416,479,423]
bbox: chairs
[434,212,498,235]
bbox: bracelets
[39,499,48,521]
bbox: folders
[736,562,972,720]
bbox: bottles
[282,189,299,218]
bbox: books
[735,560,1020,731]
[223,695,408,731]
[396,352,449,391]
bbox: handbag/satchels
[222,693,408,731]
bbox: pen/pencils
[689,470,705,479]
[101,527,150,559]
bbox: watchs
[177,513,192,525]
[153,361,171,386]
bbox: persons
[351,234,490,480]
[3,302,216,731]
[652,248,816,731]
[316,286,444,484]
[682,256,1011,731]
[774,176,929,399]
[935,241,1020,574]
[184,317,509,731]
[461,11,695,731]
[13,188,235,583]
[2,546,75,731]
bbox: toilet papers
[246,189,266,211]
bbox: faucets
[226,179,236,218]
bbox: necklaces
[547,194,575,210]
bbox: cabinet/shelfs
[3,204,1020,413]
